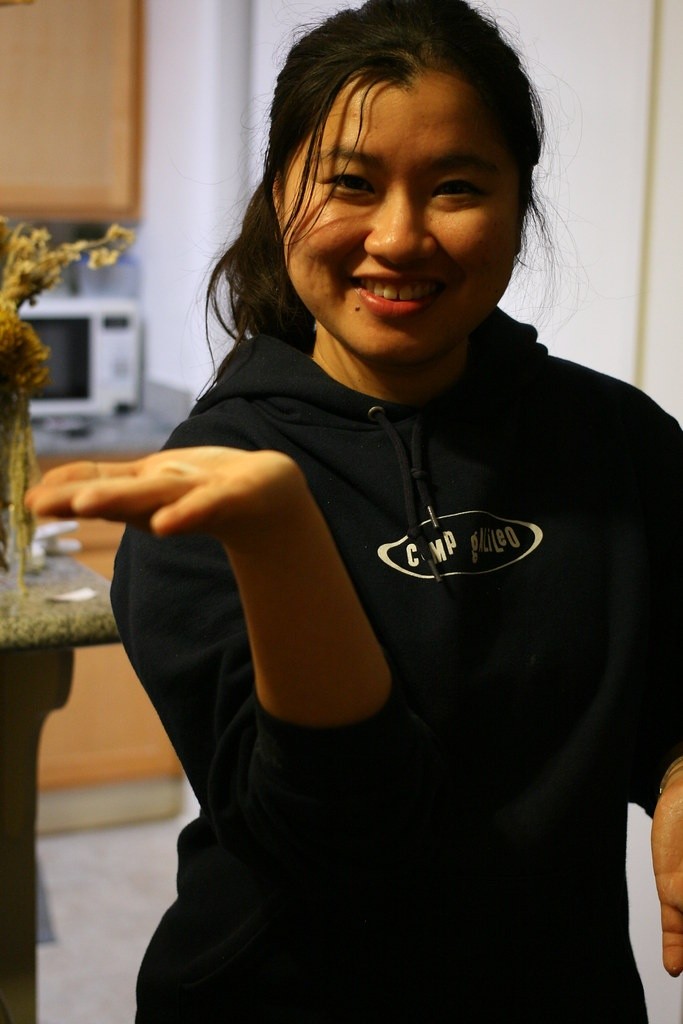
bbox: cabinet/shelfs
[2,0,144,226]
[33,476,187,833]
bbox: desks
[0,550,126,1023]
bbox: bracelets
[658,757,683,797]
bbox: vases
[0,396,38,590]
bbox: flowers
[1,217,134,415]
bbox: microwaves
[13,295,142,418]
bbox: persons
[21,0,683,1024]
